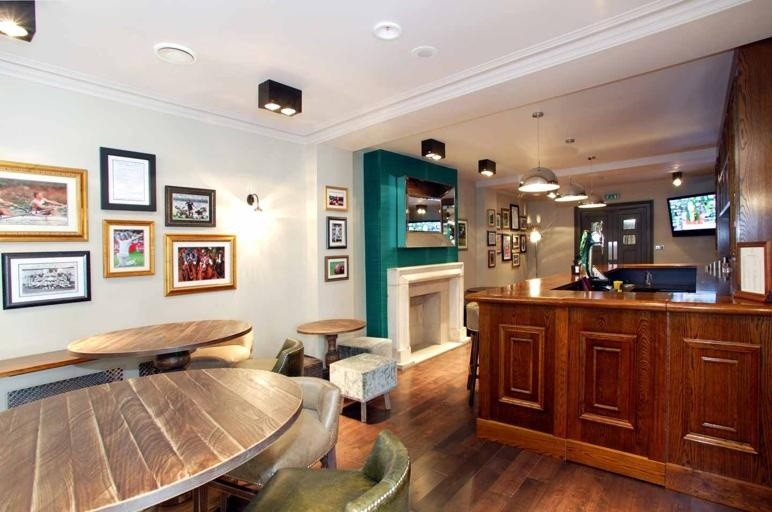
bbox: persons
[114,233,143,266]
[334,225,341,241]
[29,191,63,216]
[683,199,702,224]
[175,200,209,219]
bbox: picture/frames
[458,218,468,250]
[103,218,155,277]
[100,146,157,212]
[2,251,92,309]
[165,185,215,228]
[164,232,237,296]
[486,204,527,269]
[324,184,350,282]
[0,161,89,242]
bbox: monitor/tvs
[408,220,442,233]
[667,191,717,237]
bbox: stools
[463,286,498,407]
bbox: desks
[67,319,250,372]
[0,369,302,512]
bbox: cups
[613,281,623,293]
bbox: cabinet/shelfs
[715,55,771,302]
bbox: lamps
[478,159,496,176]
[554,137,588,203]
[527,225,543,278]
[422,139,445,161]
[0,1,36,41]
[672,172,683,187]
[518,112,560,193]
[258,80,302,117]
[577,156,607,208]
